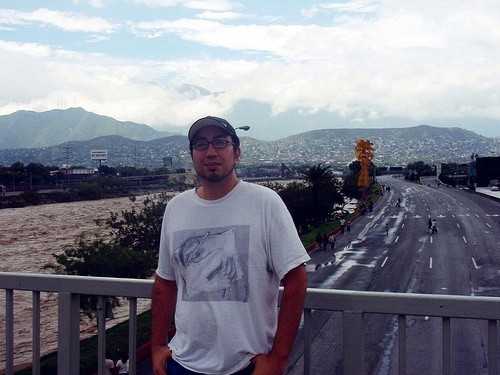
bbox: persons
[104,351,131,375]
[150,116,312,375]
[314,181,441,252]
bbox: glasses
[192,136,235,150]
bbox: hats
[188,115,240,146]
